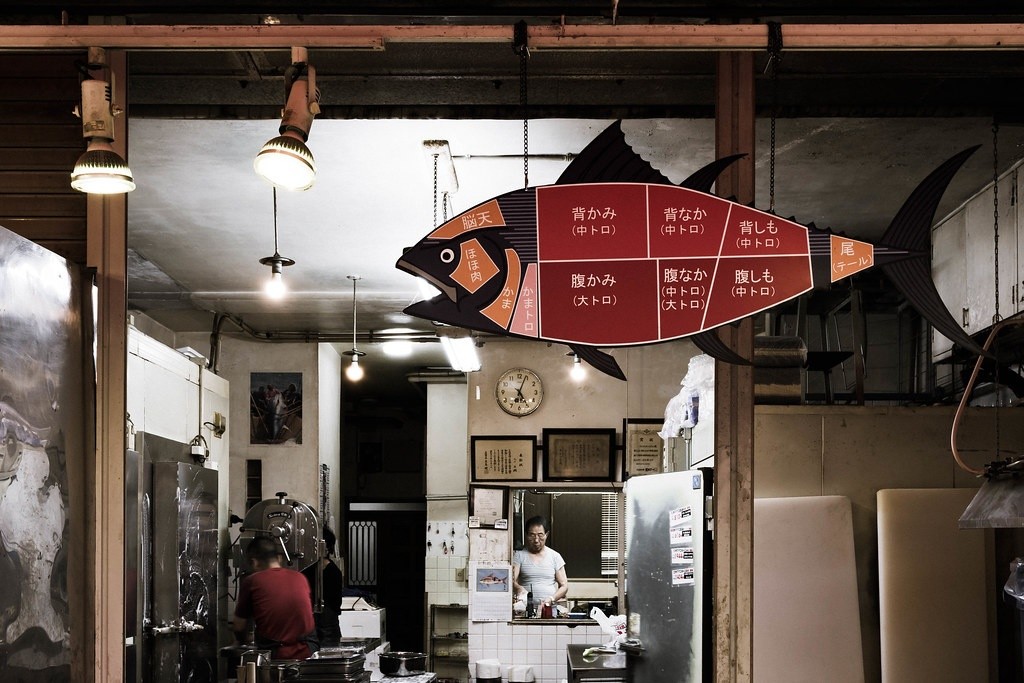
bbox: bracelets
[550,596,555,606]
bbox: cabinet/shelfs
[429,603,469,673]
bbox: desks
[566,643,627,683]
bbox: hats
[246,535,279,561]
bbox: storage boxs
[341,607,387,645]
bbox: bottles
[545,600,558,619]
[247,662,256,683]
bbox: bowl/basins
[568,613,587,618]
[378,651,426,676]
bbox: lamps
[423,139,486,373]
[71,47,136,195]
[252,45,319,194]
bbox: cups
[237,665,246,682]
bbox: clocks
[495,367,544,417]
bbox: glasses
[526,532,547,541]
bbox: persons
[232,536,319,661]
[511,515,568,608]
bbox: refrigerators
[623,470,706,683]
